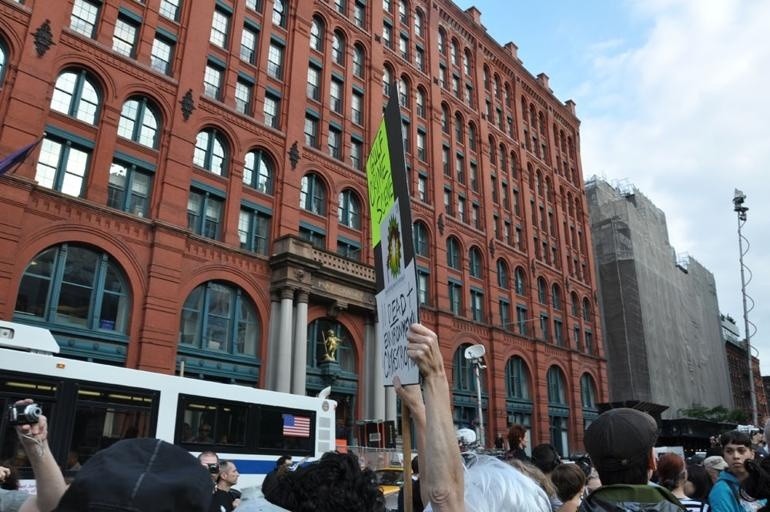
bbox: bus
[0,319,339,512]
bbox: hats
[50,439,213,511]
[583,407,660,471]
[456,426,484,453]
[531,445,561,474]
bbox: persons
[393,323,552,512]
[458,408,769,512]
[324,329,343,359]
[0,399,424,512]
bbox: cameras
[7,403,42,426]
[209,463,219,474]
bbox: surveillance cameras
[734,197,748,220]
